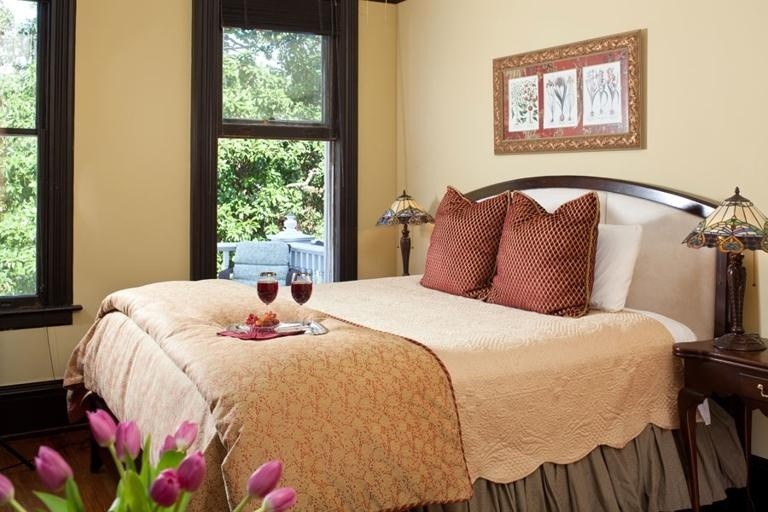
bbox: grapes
[245,311,280,327]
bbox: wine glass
[256,270,280,327]
[290,272,313,328]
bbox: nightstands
[669,338,768,512]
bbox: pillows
[417,185,643,318]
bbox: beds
[63,173,731,512]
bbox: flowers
[0,407,296,512]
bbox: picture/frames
[491,28,642,156]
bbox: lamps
[680,185,768,351]
[374,190,436,276]
